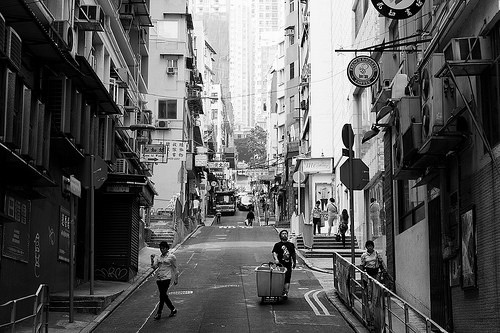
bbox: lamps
[362,123,388,144]
[2,142,20,153]
[377,98,397,121]
[20,154,36,166]
[74,143,86,151]
[65,132,75,141]
[34,165,48,174]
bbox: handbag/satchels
[244,218,249,226]
[336,230,341,241]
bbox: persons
[351,239,387,303]
[312,200,321,235]
[369,198,381,239]
[325,198,338,236]
[215,211,222,224]
[246,209,254,226]
[338,209,349,248]
[264,206,270,226]
[260,197,266,213]
[150,241,179,319]
[272,229,296,298]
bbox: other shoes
[154,313,161,319]
[169,309,177,317]
[283,291,288,297]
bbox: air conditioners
[118,67,129,87]
[116,158,129,174]
[78,4,105,31]
[0,12,24,73]
[442,36,493,61]
[166,67,175,74]
[120,4,135,17]
[287,29,295,35]
[155,119,171,128]
[191,90,198,98]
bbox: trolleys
[255,261,288,304]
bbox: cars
[237,202,254,211]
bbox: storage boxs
[255,265,287,297]
